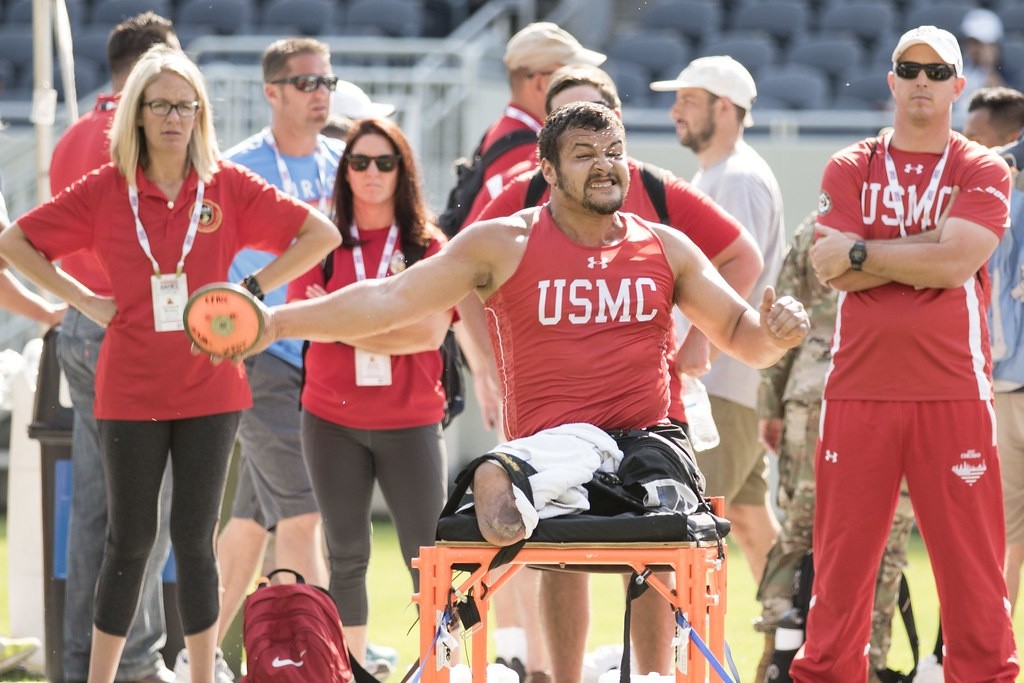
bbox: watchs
[245,275,265,302]
[849,240,867,271]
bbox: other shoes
[174,648,236,683]
[365,659,393,681]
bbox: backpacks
[239,569,378,683]
[438,130,538,240]
[297,237,474,430]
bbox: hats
[650,55,757,110]
[504,22,608,68]
[961,8,1003,41]
[892,26,963,77]
[331,78,397,121]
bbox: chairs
[0,0,1024,115]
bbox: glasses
[272,74,339,92]
[897,62,956,82]
[142,99,199,117]
[346,154,400,173]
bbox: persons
[155,34,394,683]
[438,18,607,682]
[1,190,64,328]
[790,23,1020,683]
[50,9,180,683]
[944,5,1013,138]
[287,115,471,683]
[955,85,1024,616]
[320,74,400,141]
[749,207,918,682]
[1,47,343,683]
[649,53,790,683]
[188,101,813,548]
[464,64,764,683]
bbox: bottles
[764,608,805,683]
[678,372,720,451]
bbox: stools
[409,497,729,683]
